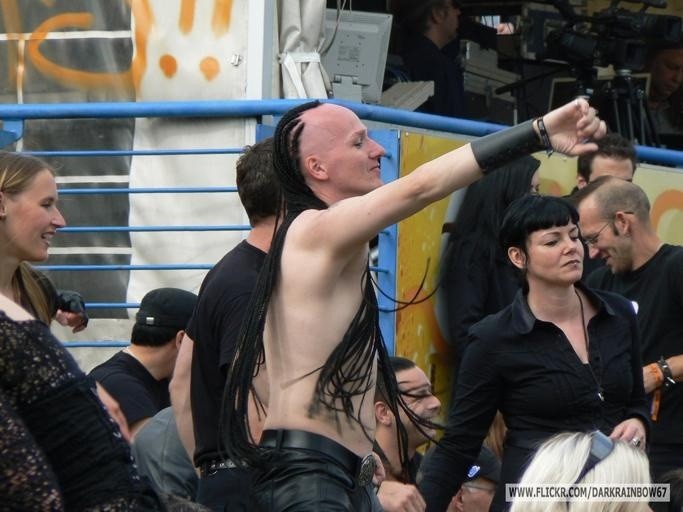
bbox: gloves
[54,289,88,334]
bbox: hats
[417,443,502,484]
[135,287,198,329]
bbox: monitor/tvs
[323,7,395,101]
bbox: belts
[257,429,376,487]
[199,458,250,470]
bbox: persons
[214,93,608,510]
[336,1,682,152]
[2,133,683,511]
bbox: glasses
[582,211,635,248]
[565,429,615,512]
